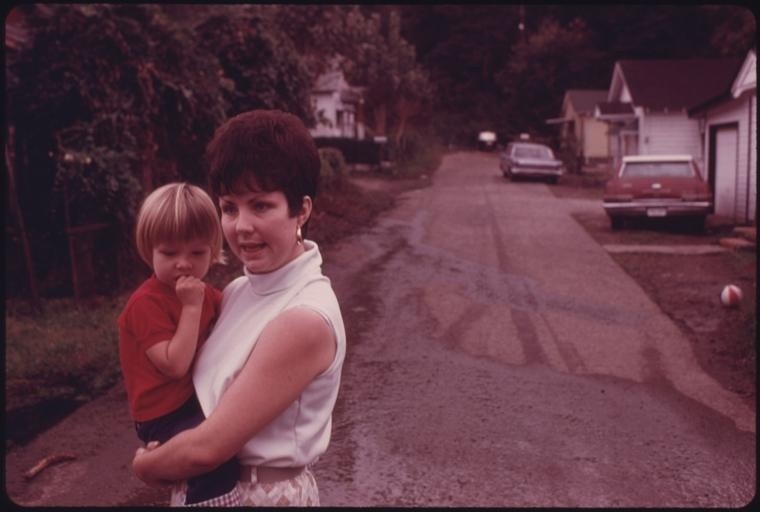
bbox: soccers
[721,284,742,306]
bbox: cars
[499,131,565,184]
[477,129,498,150]
[600,151,716,235]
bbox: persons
[115,180,238,512]
[133,108,348,508]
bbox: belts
[235,465,305,483]
